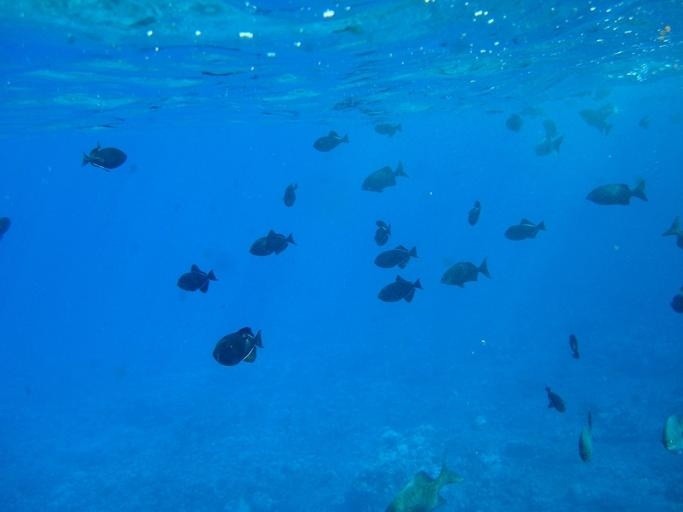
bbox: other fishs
[314,103,682,314]
